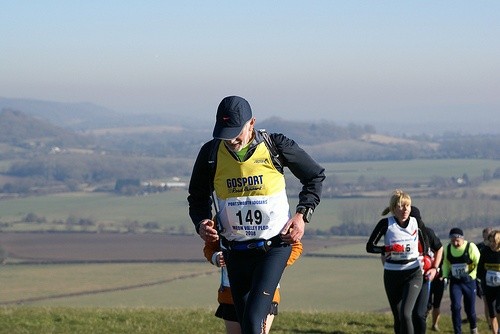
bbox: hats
[449,228,464,238]
[212,95,253,141]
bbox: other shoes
[432,325,440,331]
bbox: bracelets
[432,266,440,277]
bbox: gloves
[385,244,402,252]
[477,285,482,298]
[462,274,472,282]
[421,256,431,270]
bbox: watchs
[296,207,313,223]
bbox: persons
[381,205,444,334]
[425,250,445,332]
[478,230,500,334]
[366,191,432,334]
[476,227,494,329]
[441,228,481,334]
[204,212,303,334]
[187,95,326,334]
